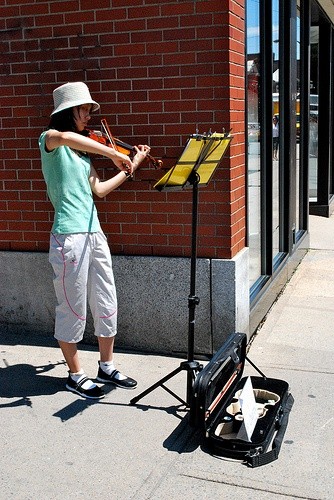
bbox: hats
[50,82,100,117]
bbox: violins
[78,127,164,171]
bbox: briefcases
[193,332,295,469]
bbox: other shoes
[97,360,138,389]
[65,370,105,399]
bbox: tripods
[130,135,234,410]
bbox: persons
[36,82,152,401]
[309,114,318,158]
[272,115,280,160]
